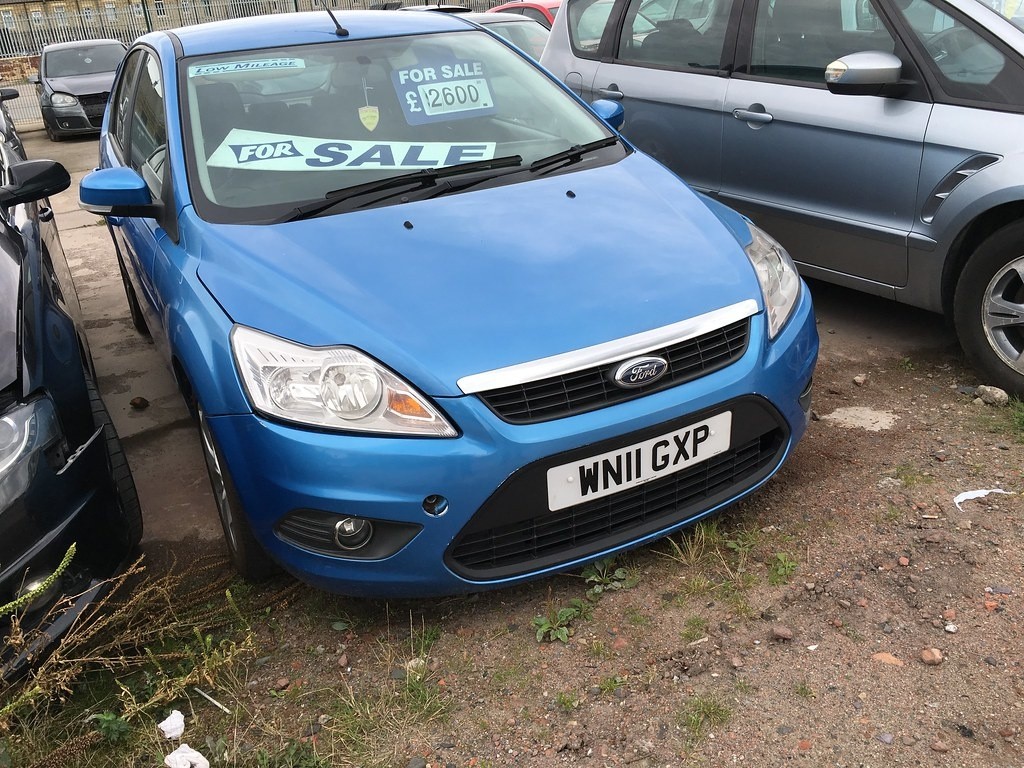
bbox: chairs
[764,0,834,84]
[639,19,717,72]
[196,83,270,202]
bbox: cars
[453,13,550,62]
[79,10,821,599]
[537,0,1024,402]
[0,144,145,703]
[0,88,29,160]
[397,5,474,14]
[485,0,660,51]
[35,39,129,142]
